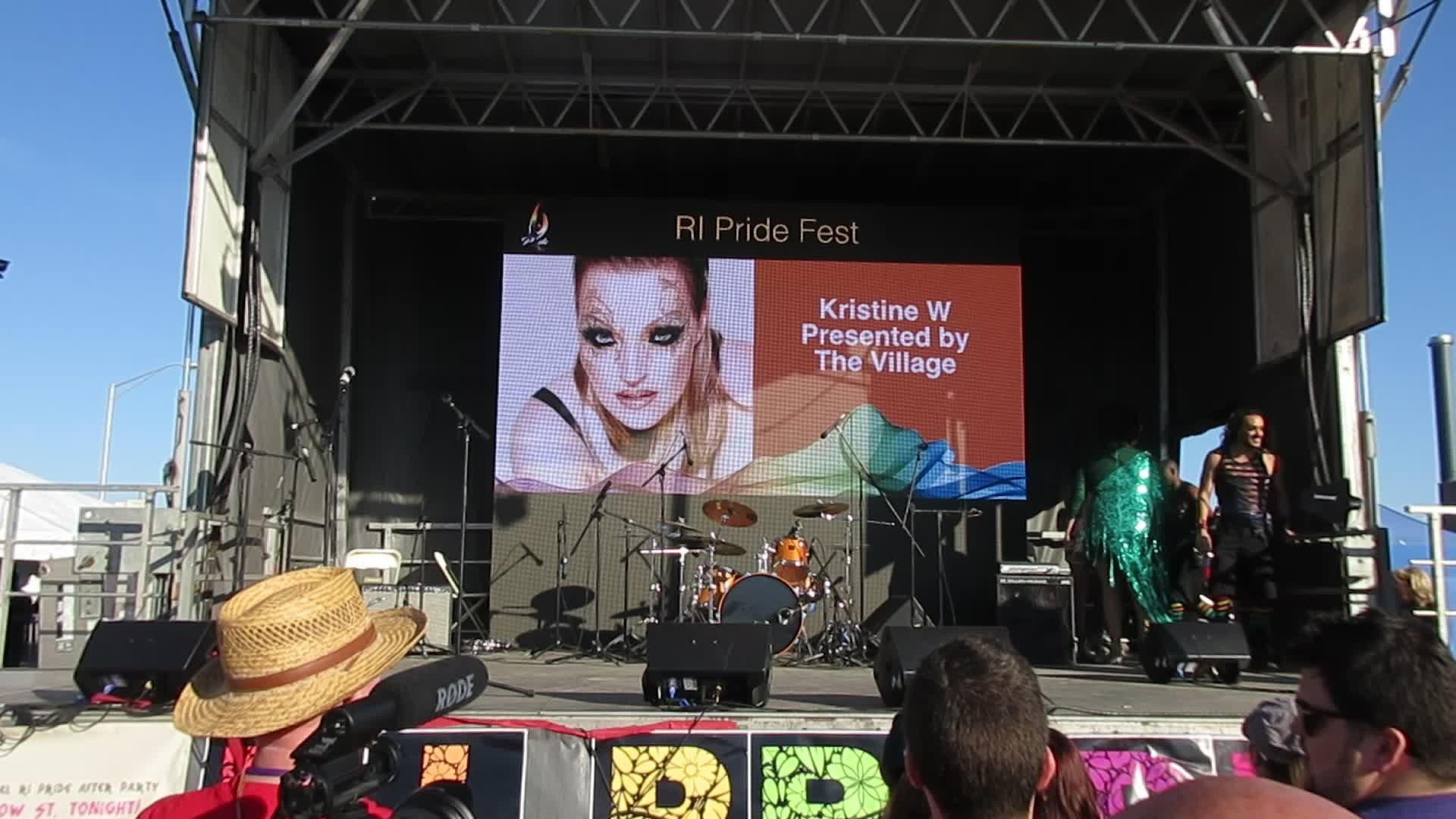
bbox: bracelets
[1198,524,1208,531]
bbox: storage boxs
[979,561,1077,665]
[359,582,453,657]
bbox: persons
[135,566,430,818]
[1240,693,1310,790]
[1196,409,1299,672]
[510,255,754,493]
[1161,459,1221,599]
[1287,608,1456,819]
[1390,564,1439,637]
[879,634,1099,819]
[1107,776,1365,818]
[1065,430,1183,665]
[4,570,32,667]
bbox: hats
[1242,698,1304,764]
[171,565,429,739]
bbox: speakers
[644,620,773,707]
[73,619,218,702]
[1139,621,1250,687]
[873,625,1008,701]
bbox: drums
[771,535,809,586]
[689,564,741,612]
[718,572,803,659]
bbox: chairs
[344,547,404,587]
[434,549,492,641]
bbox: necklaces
[244,766,292,776]
[581,391,687,476]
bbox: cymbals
[689,536,748,557]
[702,499,757,528]
[792,501,850,520]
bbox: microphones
[915,442,930,449]
[336,366,354,392]
[681,440,692,466]
[820,410,850,440]
